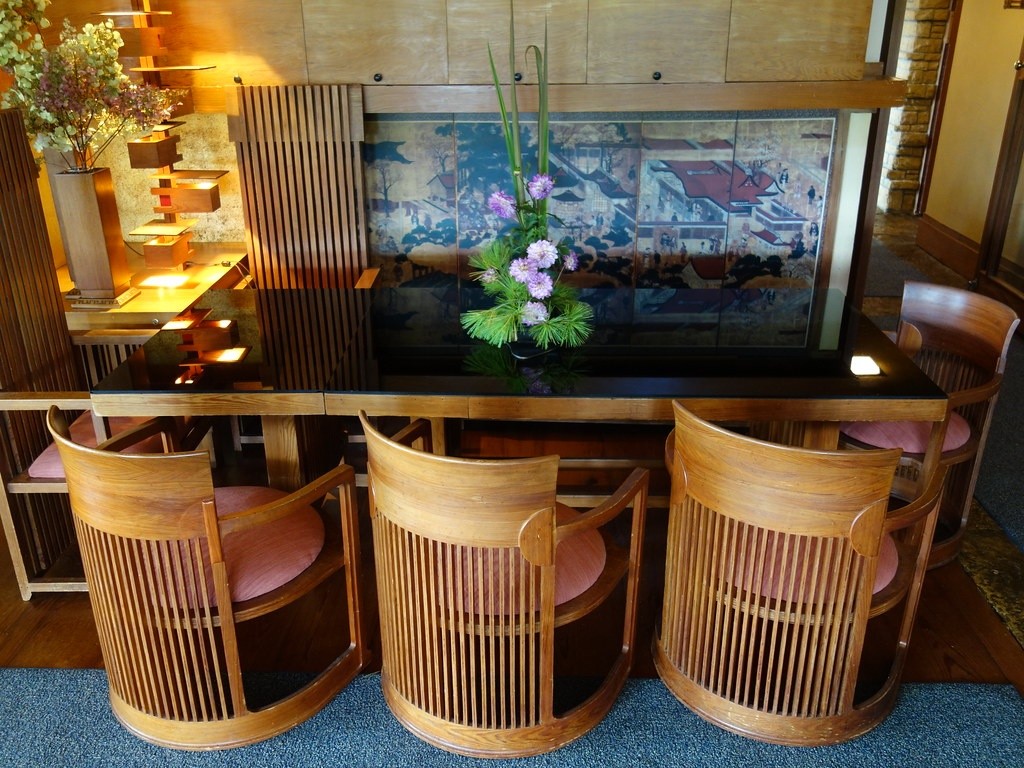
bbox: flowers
[464,344,585,398]
[0,0,186,172]
[459,0,595,351]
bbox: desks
[87,274,946,510]
[46,234,252,390]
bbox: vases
[48,164,140,309]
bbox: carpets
[0,667,1024,768]
[972,328,1024,550]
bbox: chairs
[647,397,947,748]
[830,233,1018,575]
[1,108,193,602]
[355,411,650,759]
[46,404,375,752]
